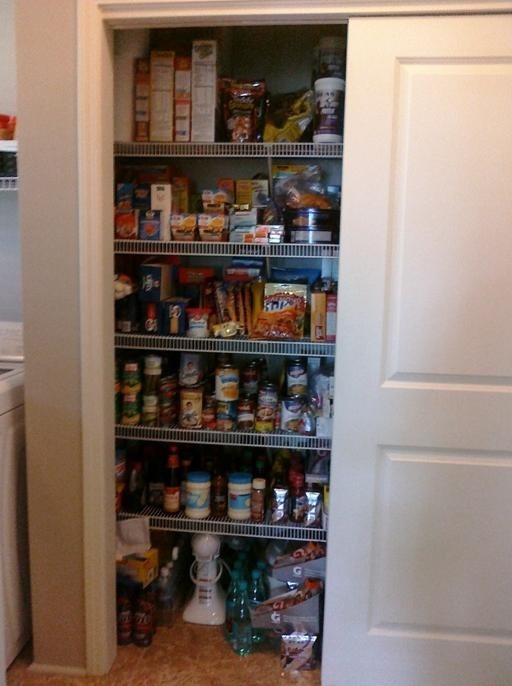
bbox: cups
[185,308,212,337]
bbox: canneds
[158,349,317,435]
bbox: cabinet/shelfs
[72,2,347,686]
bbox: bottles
[116,552,269,656]
[311,35,346,144]
[164,443,305,524]
[114,355,162,428]
